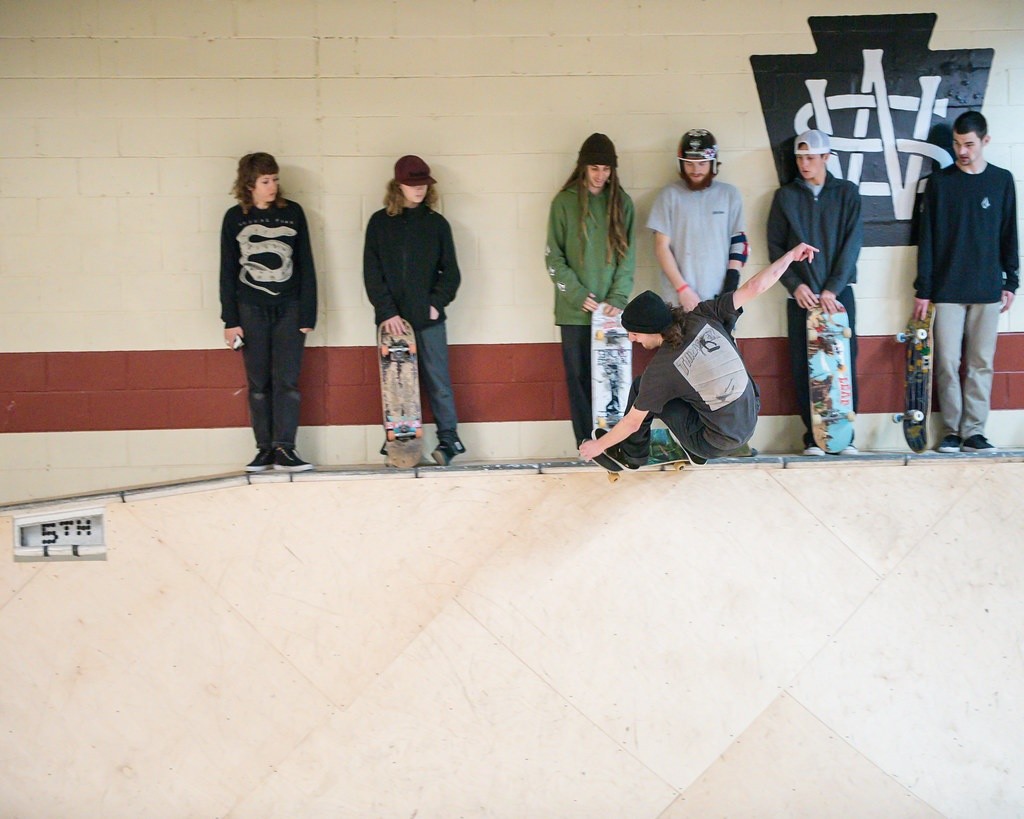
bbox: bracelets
[677,284,688,292]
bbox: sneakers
[429,438,454,465]
[963,434,998,456]
[244,447,277,473]
[667,426,708,467]
[935,433,960,453]
[271,446,314,473]
[839,443,857,457]
[590,427,642,472]
[804,441,827,457]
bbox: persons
[545,133,636,449]
[221,152,317,473]
[363,156,466,466]
[647,128,748,314]
[767,130,862,455]
[913,110,1019,454]
[579,242,820,471]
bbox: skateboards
[592,302,632,443]
[807,299,856,452]
[378,319,422,468]
[582,427,751,483]
[892,302,937,454]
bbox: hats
[794,128,840,161]
[577,132,618,168]
[392,154,437,189]
[620,287,672,336]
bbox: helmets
[676,128,720,175]
